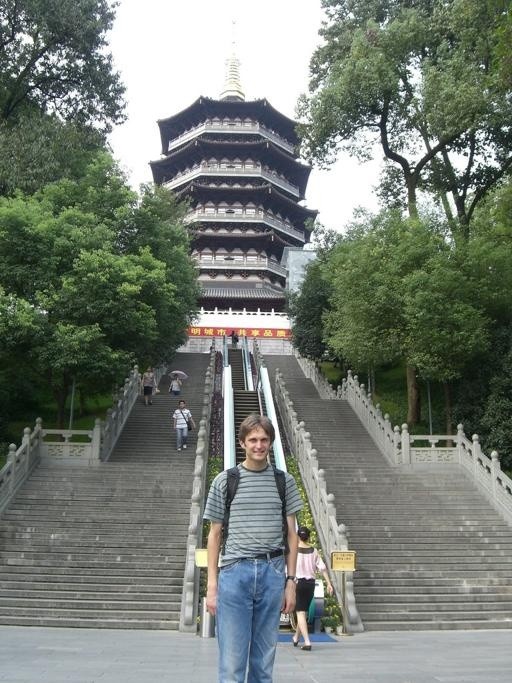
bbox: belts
[241,548,283,561]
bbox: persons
[284,526,334,650]
[227,331,239,348]
[202,412,304,683]
[172,400,196,451]
[142,366,157,406]
[169,374,183,395]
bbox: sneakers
[177,444,187,451]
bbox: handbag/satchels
[188,422,192,430]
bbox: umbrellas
[168,370,189,380]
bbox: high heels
[292,636,311,650]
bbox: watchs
[287,575,299,584]
